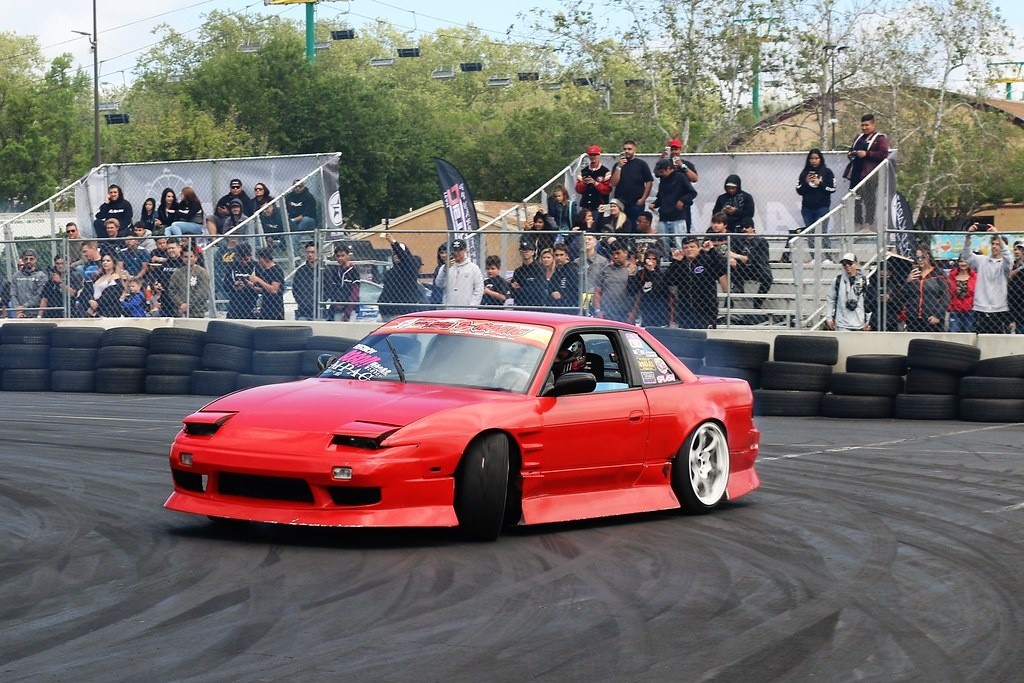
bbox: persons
[826,222,1024,332]
[494,334,587,397]
[575,141,698,263]
[376,233,511,323]
[93,178,318,245]
[842,114,888,234]
[0,218,361,322]
[510,175,774,329]
[794,148,836,265]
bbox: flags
[430,153,481,271]
[891,191,917,259]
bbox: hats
[451,239,467,249]
[587,144,600,155]
[655,159,674,169]
[609,196,625,211]
[293,178,304,186]
[838,252,857,264]
[519,240,536,250]
[668,139,682,147]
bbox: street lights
[70,30,101,167]
[822,45,850,151]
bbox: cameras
[846,299,858,310]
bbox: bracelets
[681,164,687,169]
[37,316,42,318]
[391,239,396,245]
[738,254,741,260]
[57,280,62,284]
[594,309,600,314]
[616,166,622,170]
[997,235,1002,239]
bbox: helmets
[552,334,587,377]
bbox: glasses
[254,187,263,192]
[842,261,854,266]
[914,255,928,260]
[230,185,240,189]
[66,229,75,233]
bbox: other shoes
[295,257,301,267]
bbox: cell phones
[912,264,920,278]
[665,146,671,157]
[809,171,815,179]
[382,219,395,225]
[620,152,626,157]
[600,203,611,210]
[976,224,988,231]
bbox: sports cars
[163,306,761,543]
